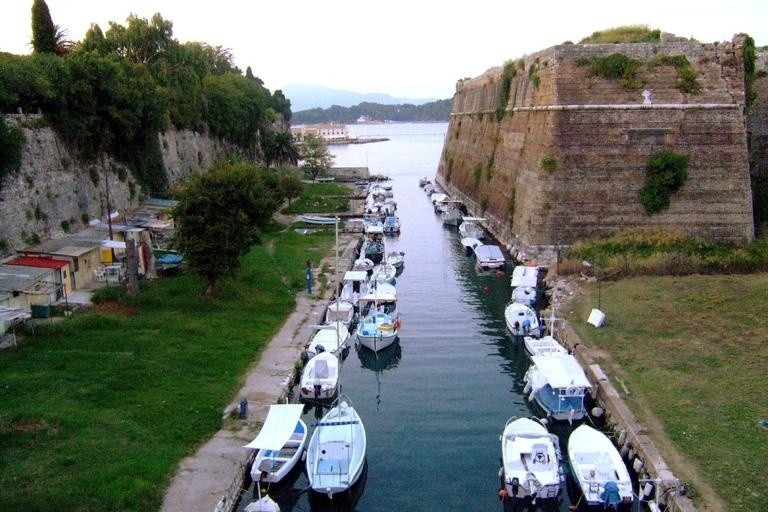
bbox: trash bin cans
[30,303,51,317]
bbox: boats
[503,300,542,339]
[418,175,486,252]
[473,244,506,270]
[154,250,184,270]
[302,215,341,224]
[305,174,405,360]
[499,417,566,506]
[298,350,341,404]
[303,393,368,498]
[517,308,594,424]
[508,264,542,306]
[241,404,309,489]
[565,422,636,507]
[522,333,569,358]
